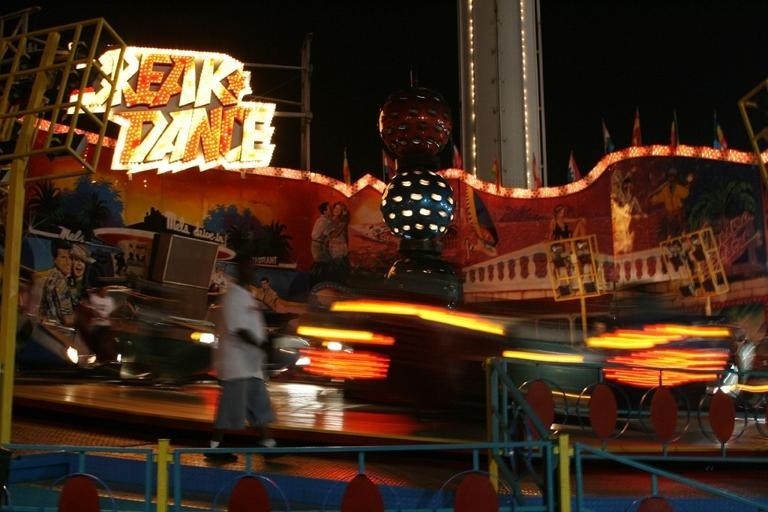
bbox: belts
[312,239,324,245]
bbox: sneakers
[263,445,289,459]
[203,445,239,463]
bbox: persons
[208,268,225,312]
[716,356,743,385]
[204,273,288,461]
[256,276,279,312]
[113,242,147,275]
[79,286,116,362]
[38,238,97,355]
[306,201,352,293]
[621,177,649,231]
[649,171,690,234]
[549,205,596,295]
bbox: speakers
[150,233,220,290]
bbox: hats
[70,243,97,264]
